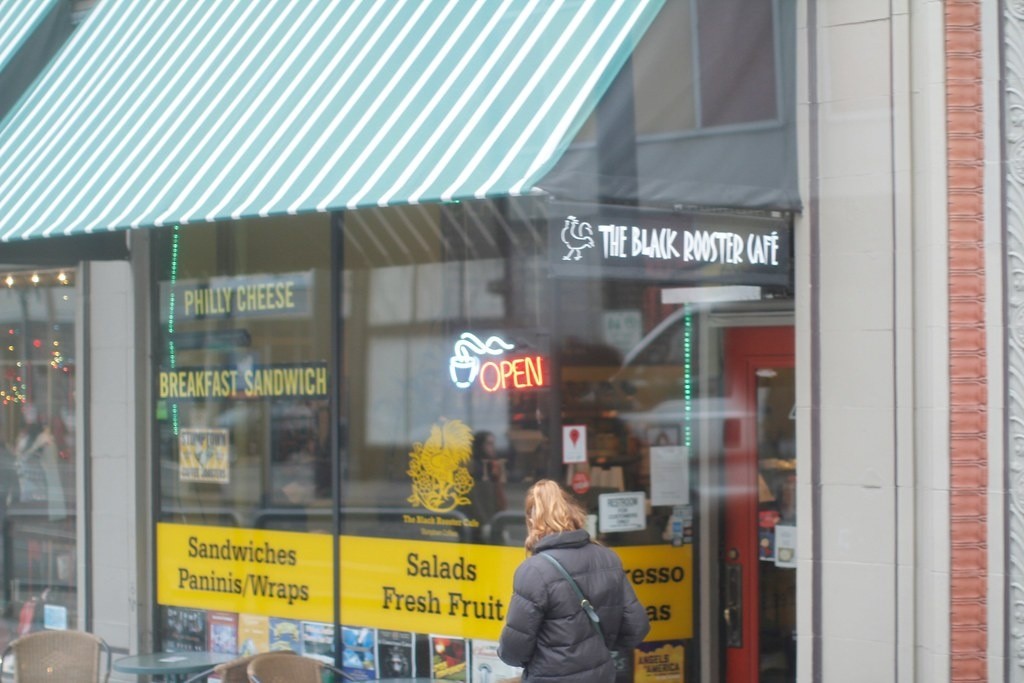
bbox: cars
[216,400,314,465]
[562,299,792,501]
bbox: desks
[114,652,241,683]
[0,629,112,683]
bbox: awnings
[0,0,669,245]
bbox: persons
[471,429,506,540]
[496,477,652,683]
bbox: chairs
[184,650,301,683]
[246,653,359,683]
